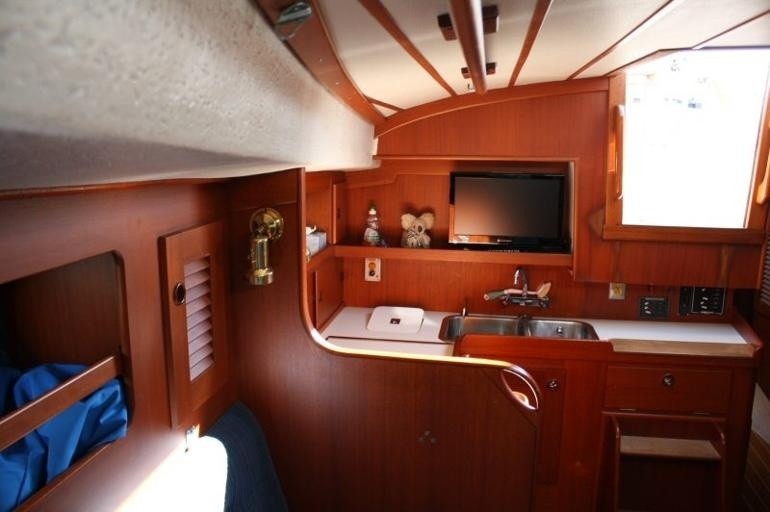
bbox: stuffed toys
[400,213,434,248]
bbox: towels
[202,400,288,512]
[305,224,326,257]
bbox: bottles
[367,208,379,232]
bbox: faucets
[513,268,528,296]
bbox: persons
[363,203,381,245]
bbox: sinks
[439,314,527,343]
[526,317,600,340]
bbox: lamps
[244,207,285,286]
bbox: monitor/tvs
[447,169,565,253]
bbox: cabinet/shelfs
[314,255,343,332]
[517,363,566,487]
[156,217,230,433]
[332,182,346,245]
